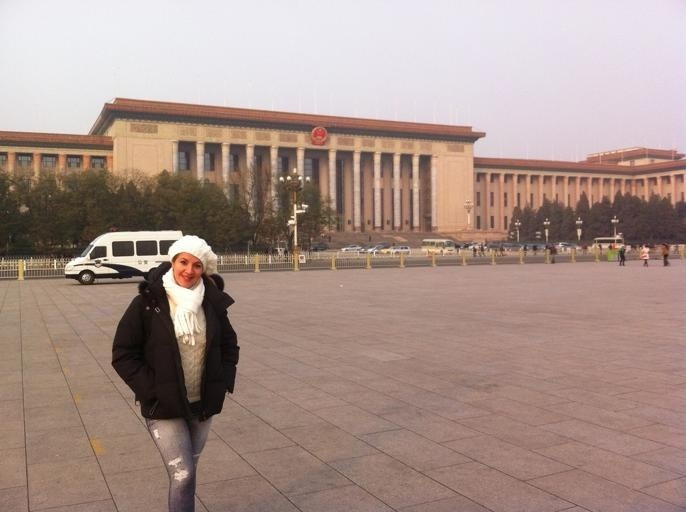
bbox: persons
[473,242,557,263]
[110,223,119,232]
[581,239,588,255]
[111,234,240,511]
[661,243,669,266]
[619,245,626,266]
[641,245,649,266]
[673,240,679,254]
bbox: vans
[64,230,183,285]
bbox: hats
[167,235,218,277]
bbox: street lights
[510,231,516,242]
[617,233,624,238]
[463,199,476,232]
[535,231,542,242]
[611,214,619,237]
[543,218,551,249]
[279,167,310,261]
[576,216,583,246]
[514,219,522,251]
